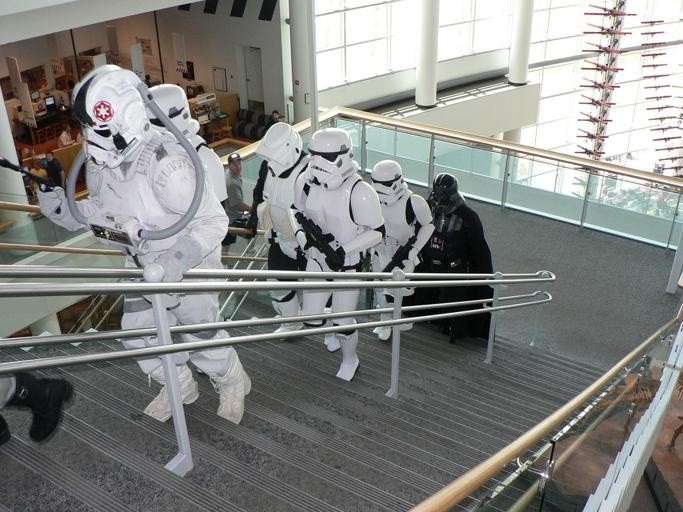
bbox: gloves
[38,186,85,231]
[154,236,201,283]
[325,246,346,271]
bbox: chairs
[211,127,223,144]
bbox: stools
[222,125,233,139]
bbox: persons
[31,66,253,426]
[58,123,75,148]
[366,160,433,342]
[40,150,66,192]
[234,123,305,340]
[220,153,255,244]
[293,130,384,380]
[138,84,226,219]
[268,110,283,125]
[409,173,493,343]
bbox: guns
[0,156,62,216]
[292,209,346,282]
[367,236,418,295]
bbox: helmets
[255,122,302,175]
[306,128,354,190]
[369,160,407,205]
[71,64,151,169]
[428,173,465,215]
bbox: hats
[229,153,241,163]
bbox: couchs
[233,108,284,141]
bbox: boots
[327,333,341,352]
[144,361,200,422]
[399,323,414,331]
[272,294,304,338]
[304,314,357,352]
[379,315,391,340]
[336,329,359,382]
[210,352,251,426]
[373,327,380,333]
[0,414,11,445]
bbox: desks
[196,112,229,137]
